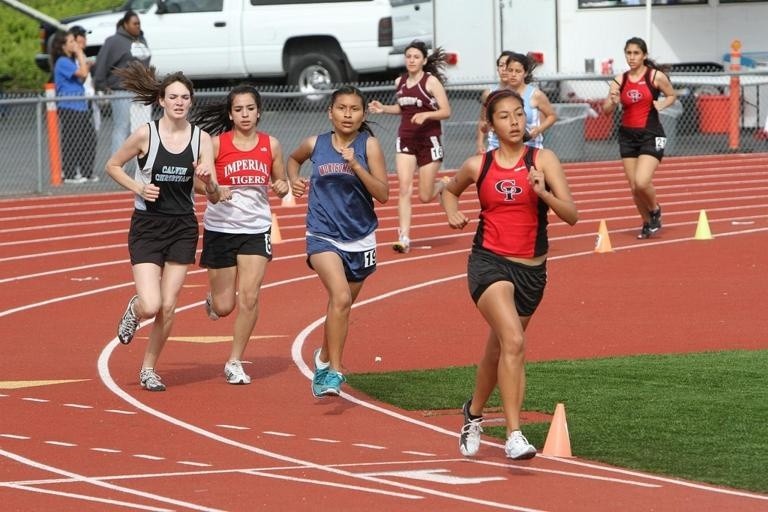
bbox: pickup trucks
[66,0,433,112]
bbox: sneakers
[88,174,99,181]
[392,235,409,253]
[648,202,660,232]
[458,400,484,456]
[61,176,88,183]
[504,430,536,460]
[320,372,346,397]
[205,293,219,321]
[139,368,165,391]
[117,294,141,345]
[223,360,252,384]
[637,223,650,239]
[311,349,329,400]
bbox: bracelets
[206,185,217,194]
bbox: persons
[91,10,152,171]
[441,89,578,458]
[69,26,101,132]
[367,38,452,254]
[487,53,557,155]
[602,37,676,240]
[51,31,99,184]
[104,59,220,392]
[286,85,389,398]
[476,50,514,155]
[194,85,289,385]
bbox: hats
[69,25,91,36]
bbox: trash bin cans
[542,102,599,163]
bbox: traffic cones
[269,211,282,244]
[591,219,614,254]
[538,402,577,459]
[691,208,714,240]
[280,178,298,207]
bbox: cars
[34,0,158,74]
